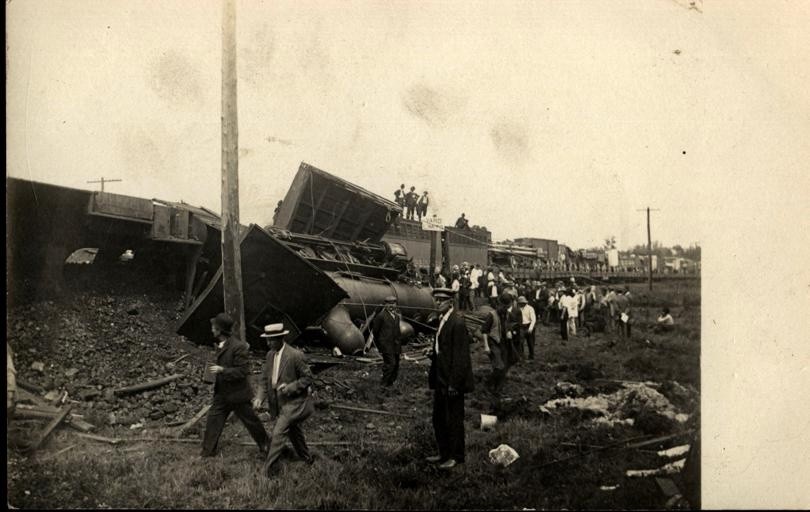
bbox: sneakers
[439,459,458,470]
[426,455,445,463]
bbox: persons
[481,294,519,400]
[421,288,472,470]
[455,213,470,229]
[419,258,633,359]
[394,184,430,223]
[372,297,402,389]
[252,322,313,479]
[199,312,272,458]
[656,309,676,332]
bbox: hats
[259,323,289,338]
[431,289,456,298]
[517,296,529,304]
[210,313,235,334]
[385,296,397,303]
[498,294,512,307]
